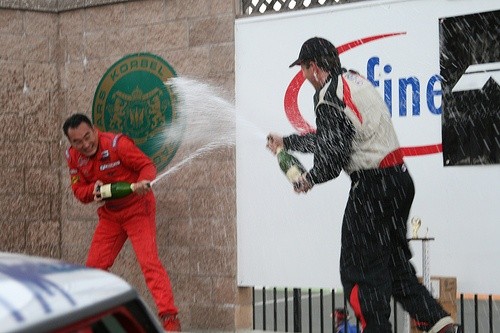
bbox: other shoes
[162,313,181,333]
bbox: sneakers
[422,316,455,333]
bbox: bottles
[267,135,311,192]
[96,181,152,202]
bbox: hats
[289,37,339,68]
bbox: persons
[61,112,182,333]
[267,37,459,333]
